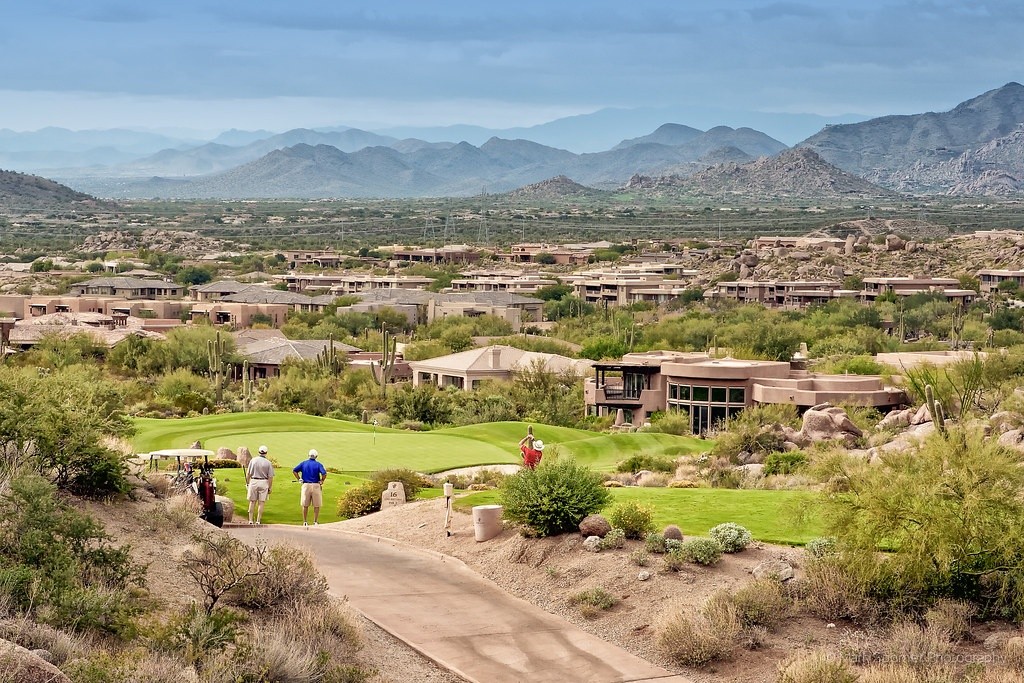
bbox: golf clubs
[291,480,300,483]
[242,464,247,485]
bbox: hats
[309,449,318,456]
[533,439,545,451]
[258,446,268,453]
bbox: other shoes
[303,522,308,526]
[255,522,260,524]
[249,521,253,524]
[313,522,319,525]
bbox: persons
[518,433,544,470]
[292,448,329,525]
[244,446,274,524]
